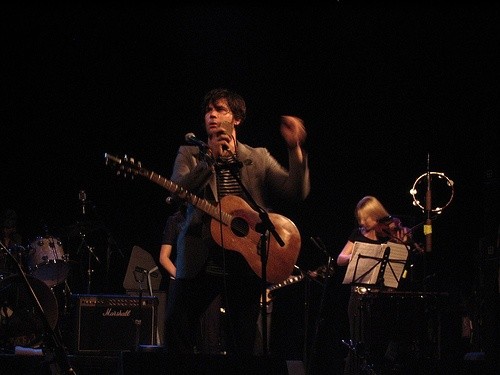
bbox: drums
[0,274,60,354]
[24,235,70,286]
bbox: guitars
[258,266,324,310]
[102,149,301,284]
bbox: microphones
[217,130,231,149]
[184,133,210,149]
[78,190,87,214]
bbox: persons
[336,196,424,375]
[159,89,310,375]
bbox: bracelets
[286,143,299,149]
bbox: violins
[375,216,422,251]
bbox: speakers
[65,293,290,375]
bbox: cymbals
[55,215,104,241]
[410,171,456,214]
